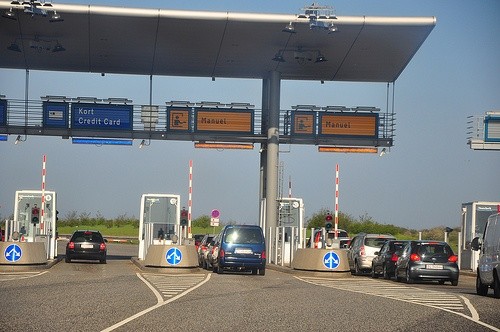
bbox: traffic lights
[325,215,333,231]
[180,210,188,226]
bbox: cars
[193,233,219,273]
[327,236,352,250]
[370,240,420,279]
[342,231,397,276]
[395,242,460,286]
[64,229,109,264]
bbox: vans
[471,205,500,298]
[218,225,266,275]
[308,229,348,248]
